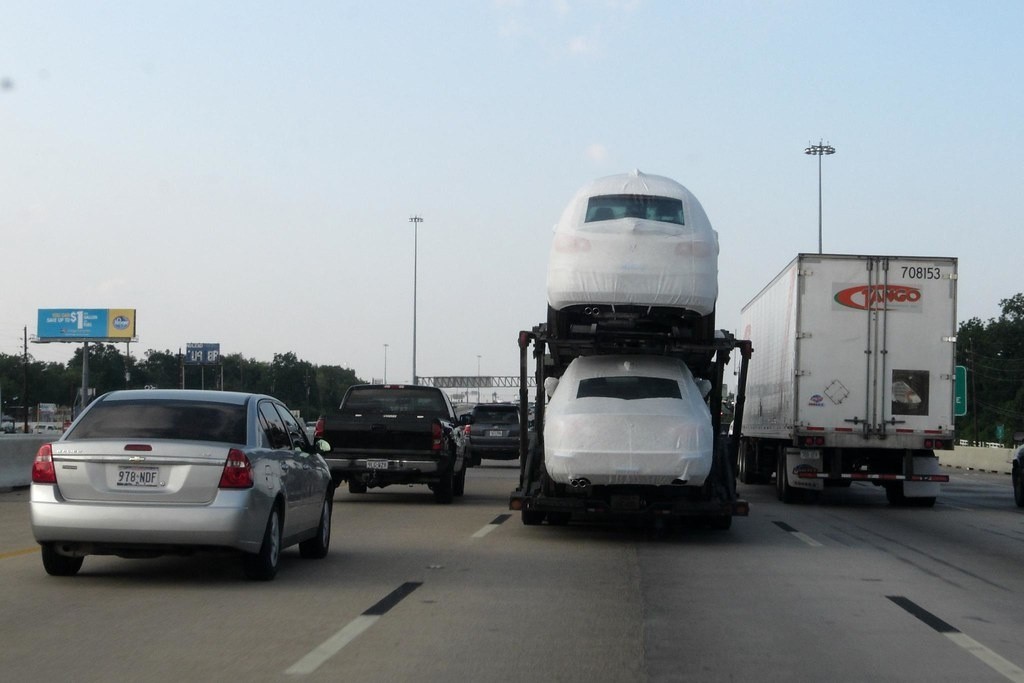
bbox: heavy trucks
[727,253,958,507]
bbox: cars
[1011,444,1024,508]
[29,390,336,581]
[543,355,713,488]
[546,168,719,342]
[33,424,56,435]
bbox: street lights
[409,215,423,385]
[476,354,482,403]
[384,343,389,384]
[805,138,835,253]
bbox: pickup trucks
[313,384,465,504]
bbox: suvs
[460,402,521,468]
